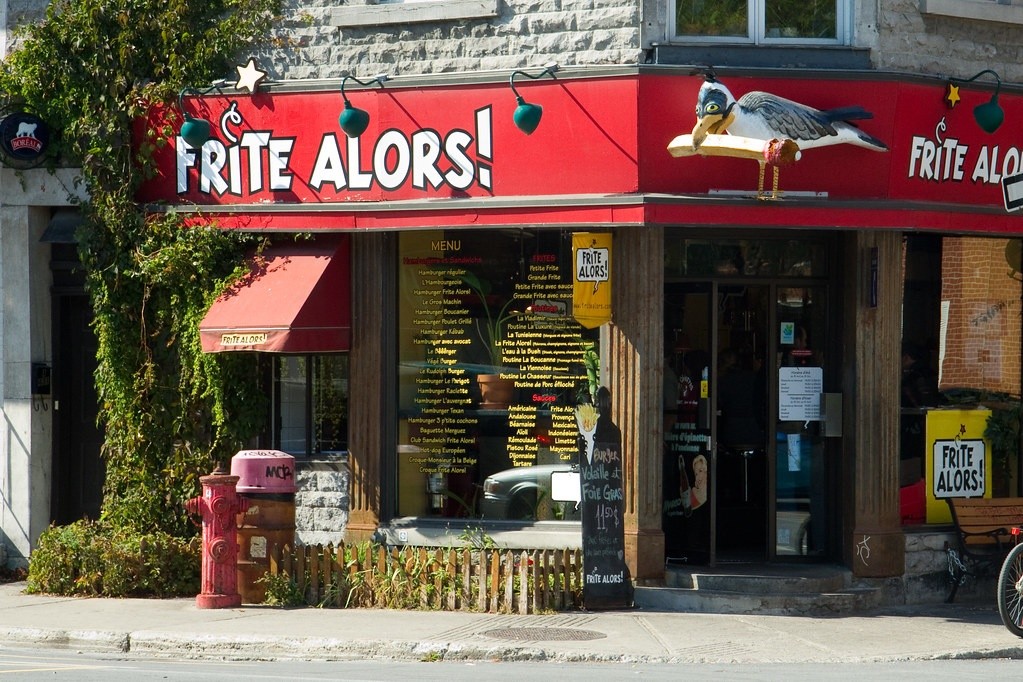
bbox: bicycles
[998,527,1023,637]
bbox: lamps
[178,79,227,149]
[338,73,390,136]
[510,61,560,135]
[942,69,1005,134]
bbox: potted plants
[427,256,600,409]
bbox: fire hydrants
[182,463,249,608]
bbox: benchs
[943,495,1023,604]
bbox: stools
[725,442,765,503]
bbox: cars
[482,464,582,520]
